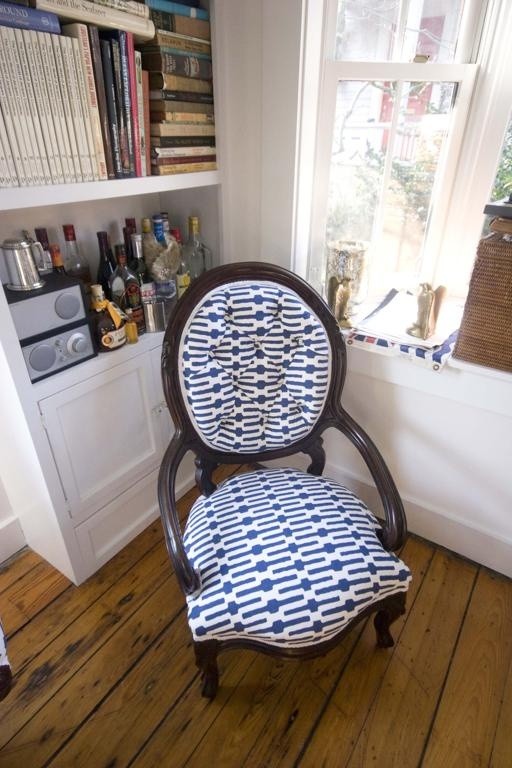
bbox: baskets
[450,231,512,371]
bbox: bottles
[34,211,210,352]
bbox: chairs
[154,261,407,695]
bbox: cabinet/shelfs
[0,163,219,587]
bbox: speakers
[0,269,100,383]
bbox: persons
[0,0,217,190]
